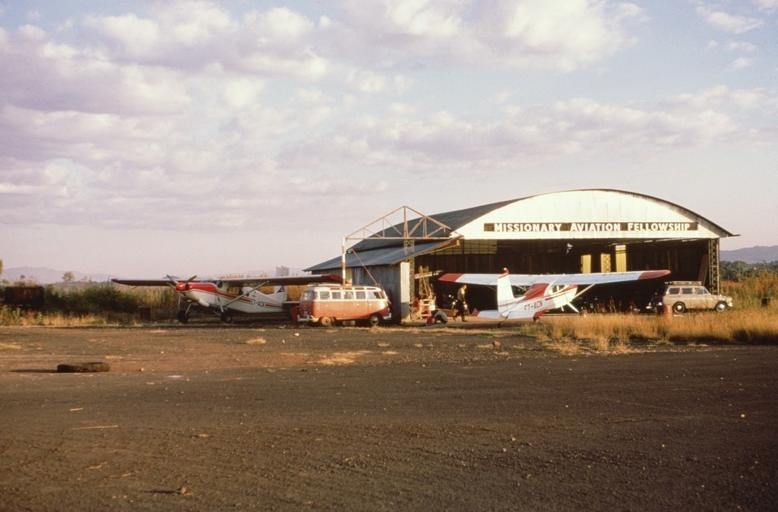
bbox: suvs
[646,281,733,314]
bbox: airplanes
[439,267,671,328]
[111,273,349,323]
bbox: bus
[297,282,392,327]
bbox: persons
[500,267,509,278]
[452,285,468,322]
